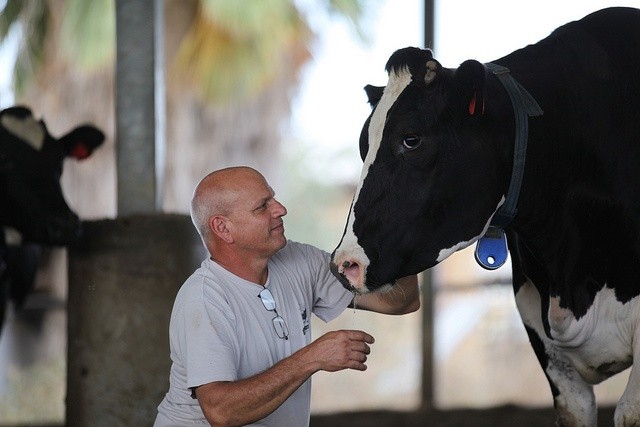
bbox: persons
[153,165,421,427]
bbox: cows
[328,2,640,427]
[0,103,106,349]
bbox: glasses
[257,285,289,341]
